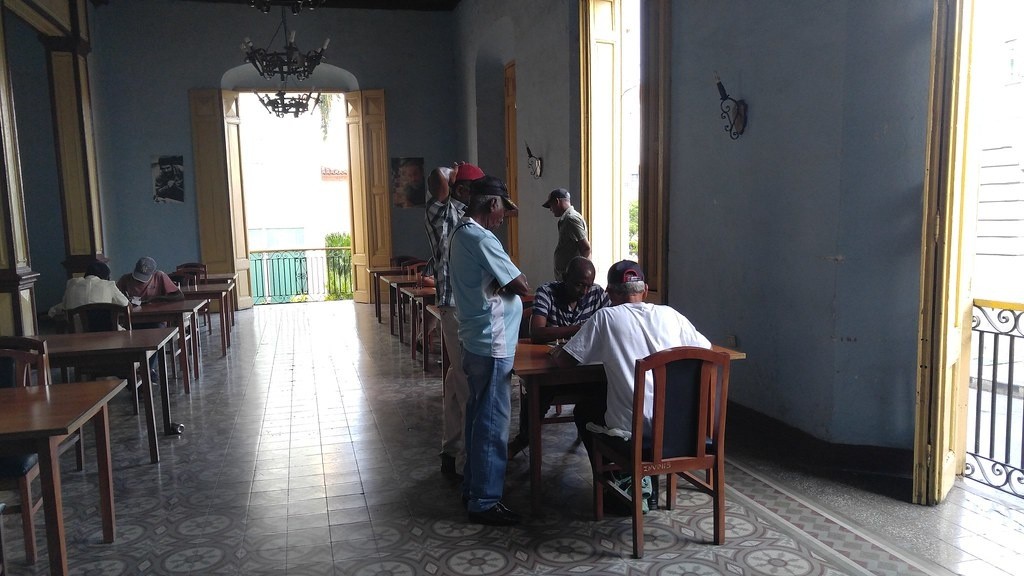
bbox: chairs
[167,269,202,348]
[176,262,210,326]
[66,303,144,417]
[0,349,49,565]
[592,346,731,560]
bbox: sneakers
[469,504,524,525]
[439,455,458,473]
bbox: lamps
[711,68,750,143]
[236,0,332,118]
[523,139,543,180]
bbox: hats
[456,163,485,180]
[542,188,570,209]
[133,257,157,281]
[605,260,645,292]
[471,175,518,210]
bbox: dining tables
[365,255,447,397]
[200,273,239,326]
[509,336,746,502]
[1,325,180,463]
[119,298,207,396]
[174,283,236,358]
[0,379,129,576]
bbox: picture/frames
[148,153,187,205]
[389,155,428,210]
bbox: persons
[507,256,613,463]
[400,161,424,208]
[61,257,150,403]
[548,260,722,519]
[541,188,592,281]
[422,158,499,489]
[113,251,187,384]
[448,175,530,527]
[153,153,183,201]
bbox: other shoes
[150,371,158,384]
[603,476,651,513]
